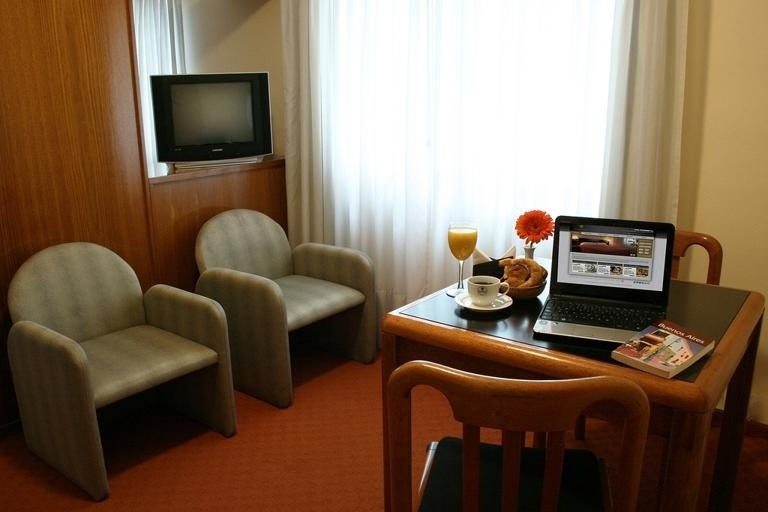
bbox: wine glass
[446,226,478,296]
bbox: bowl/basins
[505,283,547,299]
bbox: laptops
[533,216,675,345]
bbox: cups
[467,275,506,305]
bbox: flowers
[515,210,556,244]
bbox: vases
[524,245,535,259]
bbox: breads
[499,258,542,288]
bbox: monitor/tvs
[151,72,273,168]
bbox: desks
[381,254,765,512]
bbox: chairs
[381,356,652,512]
[672,230,720,286]
[193,211,379,413]
[8,239,239,501]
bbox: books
[611,318,716,380]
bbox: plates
[454,292,512,312]
